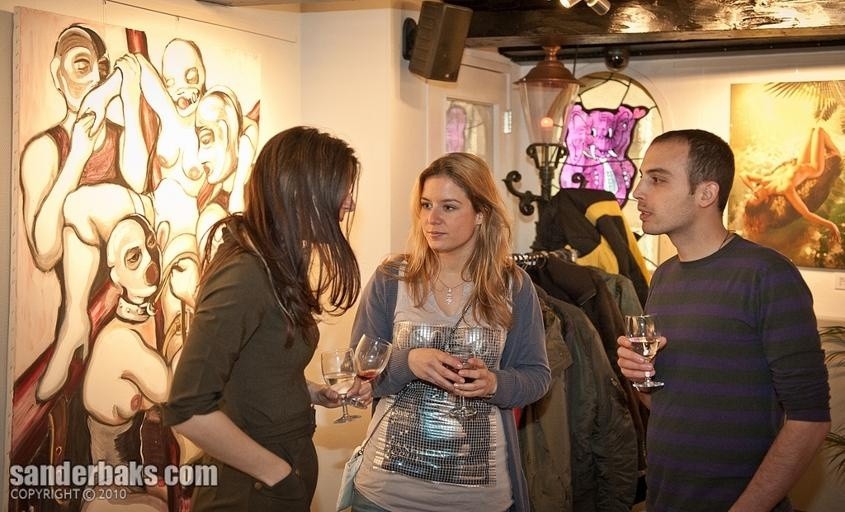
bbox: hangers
[511,249,570,278]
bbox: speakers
[408,0,474,83]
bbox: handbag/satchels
[335,442,366,512]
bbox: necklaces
[430,264,472,308]
[719,227,736,248]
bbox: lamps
[503,45,581,216]
[560,0,611,15]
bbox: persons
[616,130,832,512]
[161,125,363,512]
[335,155,551,509]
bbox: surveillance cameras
[605,48,629,67]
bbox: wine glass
[321,333,394,424]
[444,345,480,419]
[624,313,666,390]
